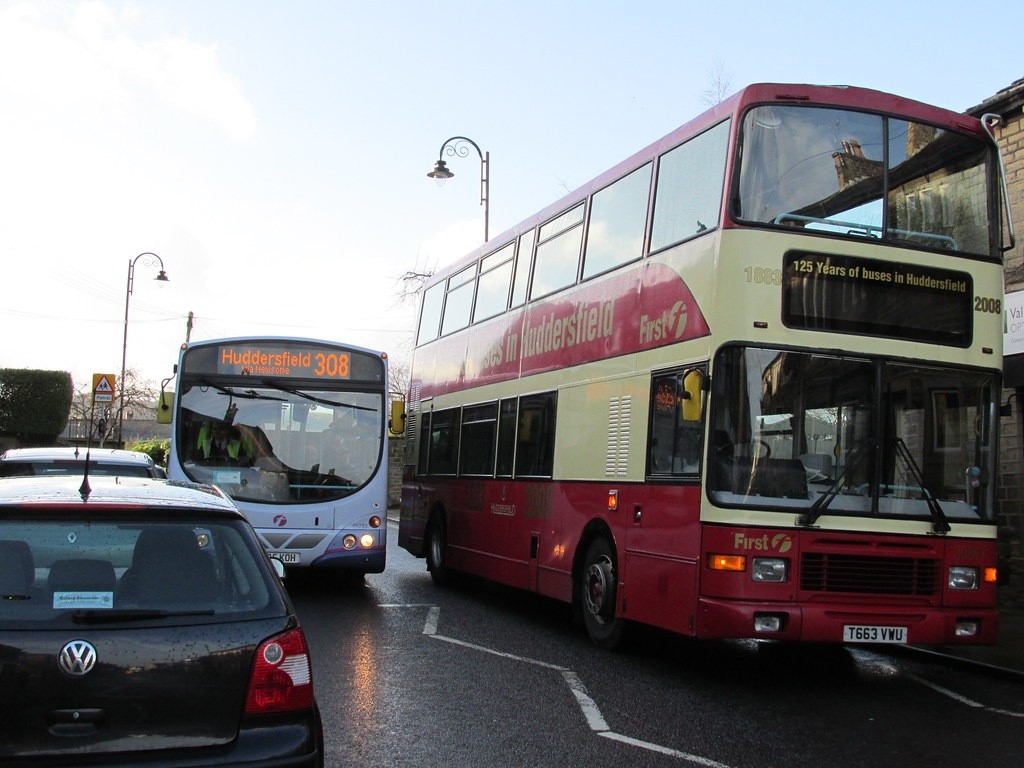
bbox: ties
[219,442,221,449]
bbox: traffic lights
[98,418,106,439]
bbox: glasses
[212,427,218,430]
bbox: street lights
[427,135,491,243]
[118,251,171,449]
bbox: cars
[0,474,325,768]
[1,444,166,479]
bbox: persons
[301,429,363,478]
[117,528,210,608]
[196,419,258,468]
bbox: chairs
[670,426,736,491]
[0,527,220,619]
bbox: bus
[397,79,1024,651]
[156,333,405,588]
